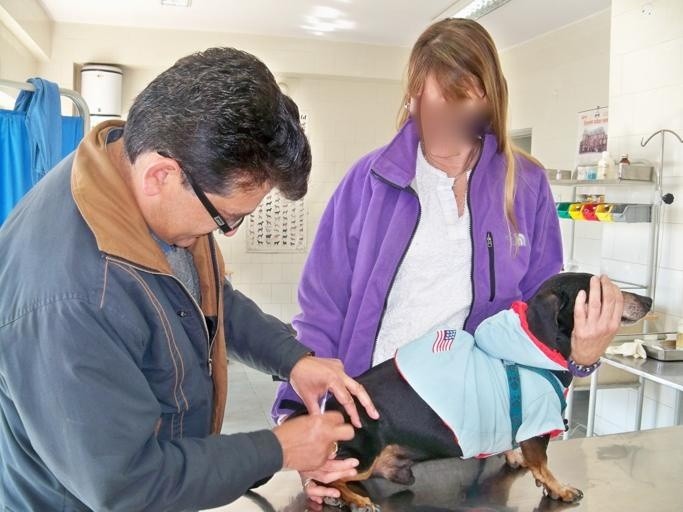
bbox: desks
[561,351,683,436]
[195,423,682,512]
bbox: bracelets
[568,359,600,372]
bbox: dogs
[250,272,653,512]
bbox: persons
[273,17,625,503]
[0,47,382,511]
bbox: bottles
[595,151,614,181]
[554,169,562,180]
[617,153,630,180]
[675,319,682,350]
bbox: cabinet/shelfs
[535,158,657,439]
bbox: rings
[331,441,338,455]
[300,477,313,488]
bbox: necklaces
[420,143,470,187]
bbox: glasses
[158,150,244,235]
